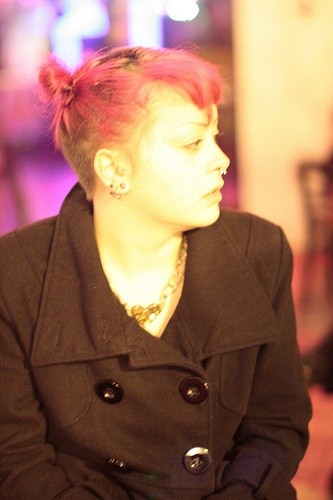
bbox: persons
[0,47,313,500]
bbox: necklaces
[103,239,188,329]
